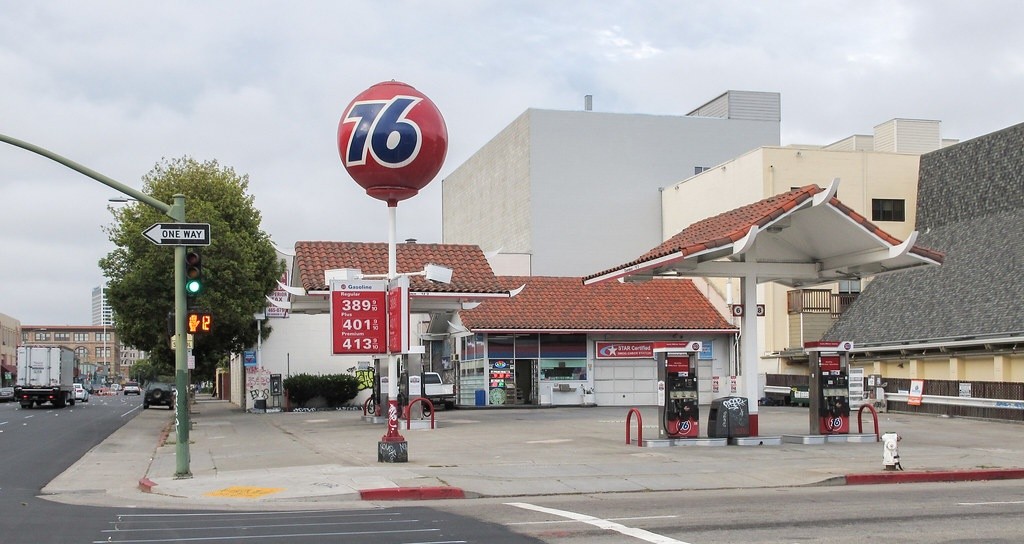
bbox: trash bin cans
[707,395,750,438]
[790,386,809,407]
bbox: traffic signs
[140,222,211,247]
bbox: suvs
[124,382,140,396]
[142,381,175,411]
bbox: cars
[0,387,17,403]
[82,384,95,396]
[97,383,122,396]
[72,383,89,402]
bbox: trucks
[16,345,77,409]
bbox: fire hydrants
[879,432,902,471]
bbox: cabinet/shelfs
[490,362,524,404]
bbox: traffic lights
[184,250,204,299]
[187,312,214,335]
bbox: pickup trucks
[425,372,457,411]
